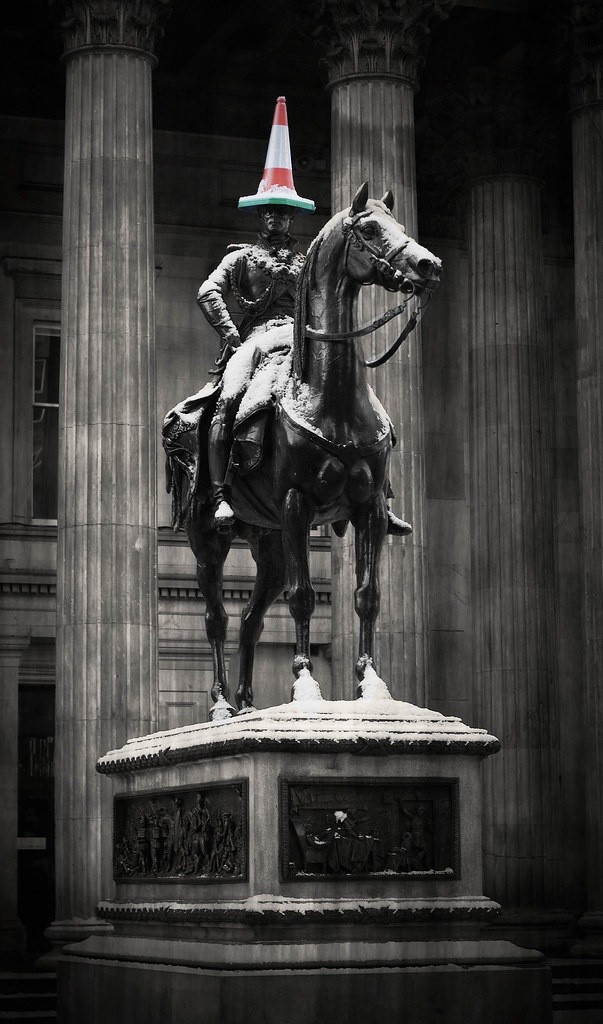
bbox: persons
[195,202,413,536]
[116,791,239,879]
[402,807,431,851]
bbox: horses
[161,181,442,721]
